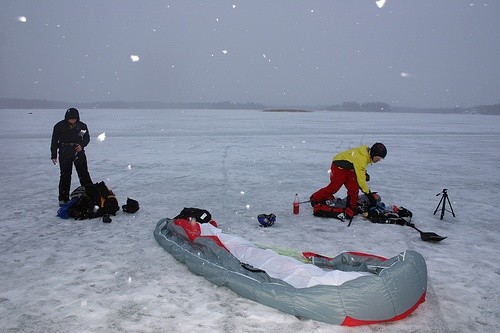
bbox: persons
[50,108,93,213]
[310,142,387,216]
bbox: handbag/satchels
[313,191,413,224]
[173,207,211,225]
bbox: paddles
[397,208,447,242]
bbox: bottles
[294,194,299,214]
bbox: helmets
[64,108,80,121]
[257,213,275,226]
[126,197,139,214]
[370,142,387,160]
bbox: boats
[152,217,428,327]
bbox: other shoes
[310,196,315,206]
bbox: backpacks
[58,181,120,223]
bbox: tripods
[433,189,455,220]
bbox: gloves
[365,190,377,205]
[366,173,370,182]
